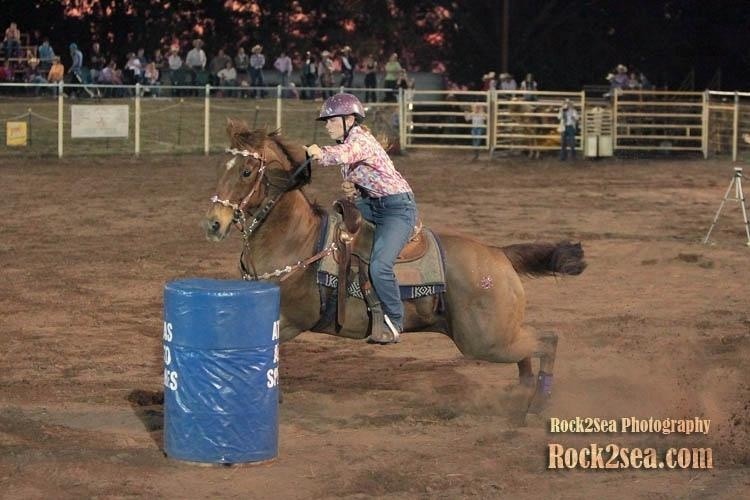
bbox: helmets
[314,91,367,123]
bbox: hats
[252,44,263,54]
[616,64,628,73]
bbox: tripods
[703,166,750,245]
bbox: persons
[301,91,419,345]
[422,59,651,164]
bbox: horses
[508,97,555,161]
[199,116,588,425]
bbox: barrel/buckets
[163,278,280,469]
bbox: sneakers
[371,316,405,342]
